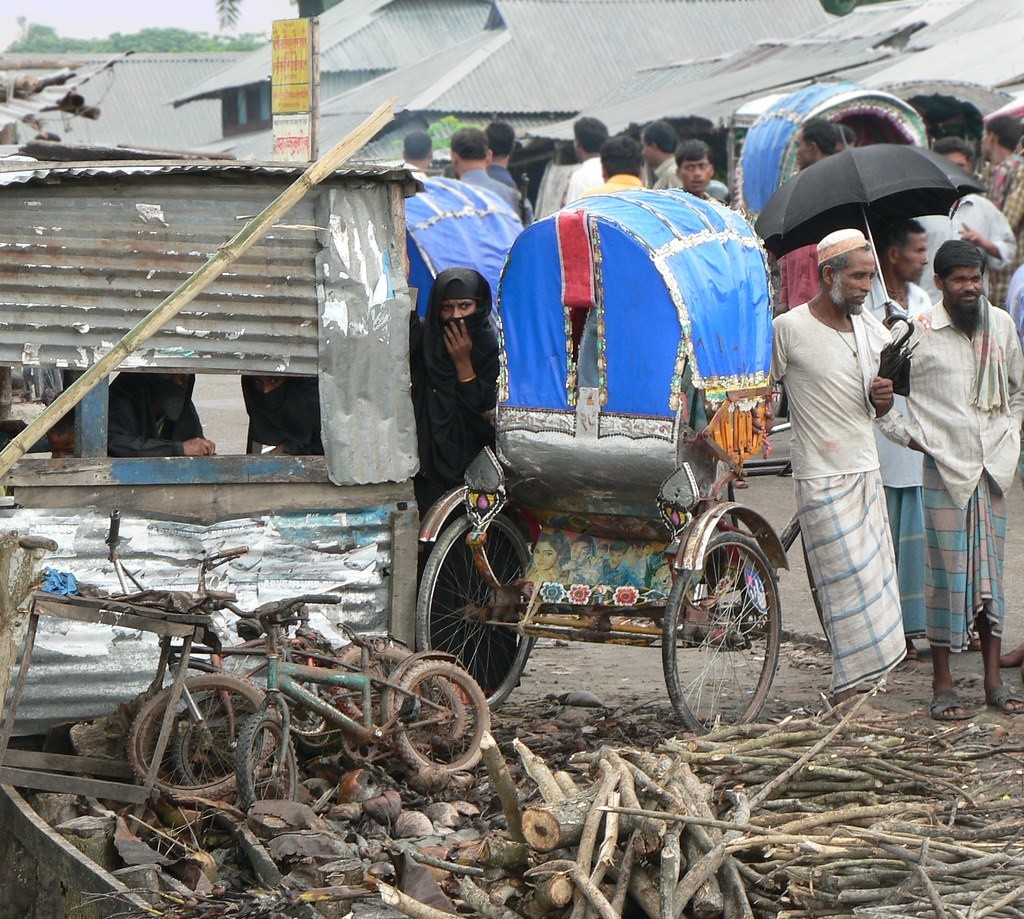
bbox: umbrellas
[868,315,917,419]
[752,142,986,307]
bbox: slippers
[929,690,969,719]
[986,684,1024,715]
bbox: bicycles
[104,508,491,819]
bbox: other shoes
[903,647,918,659]
[968,637,983,651]
[999,647,1024,667]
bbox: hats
[816,228,865,265]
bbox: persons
[402,118,742,224]
[47,372,326,458]
[766,229,907,720]
[854,218,985,659]
[793,116,1024,304]
[410,267,520,686]
[875,238,1024,720]
[1000,255,1024,674]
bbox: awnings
[520,39,1024,143]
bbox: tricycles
[415,187,829,737]
[736,81,928,416]
